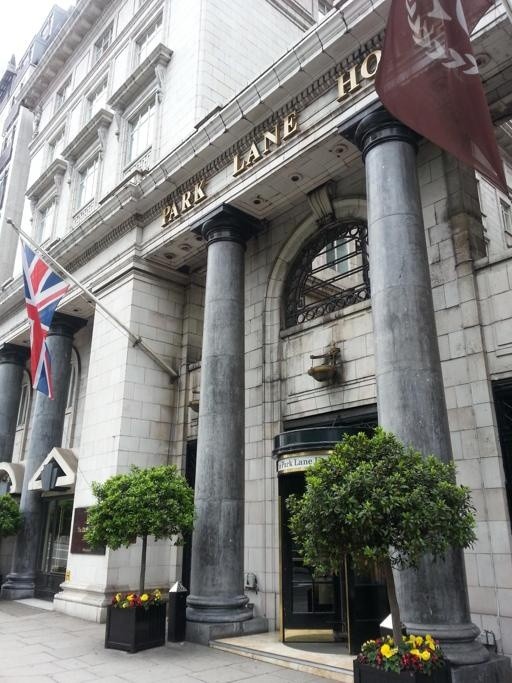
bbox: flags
[373,1,510,202]
[13,230,70,402]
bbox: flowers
[359,634,448,676]
[112,588,163,609]
